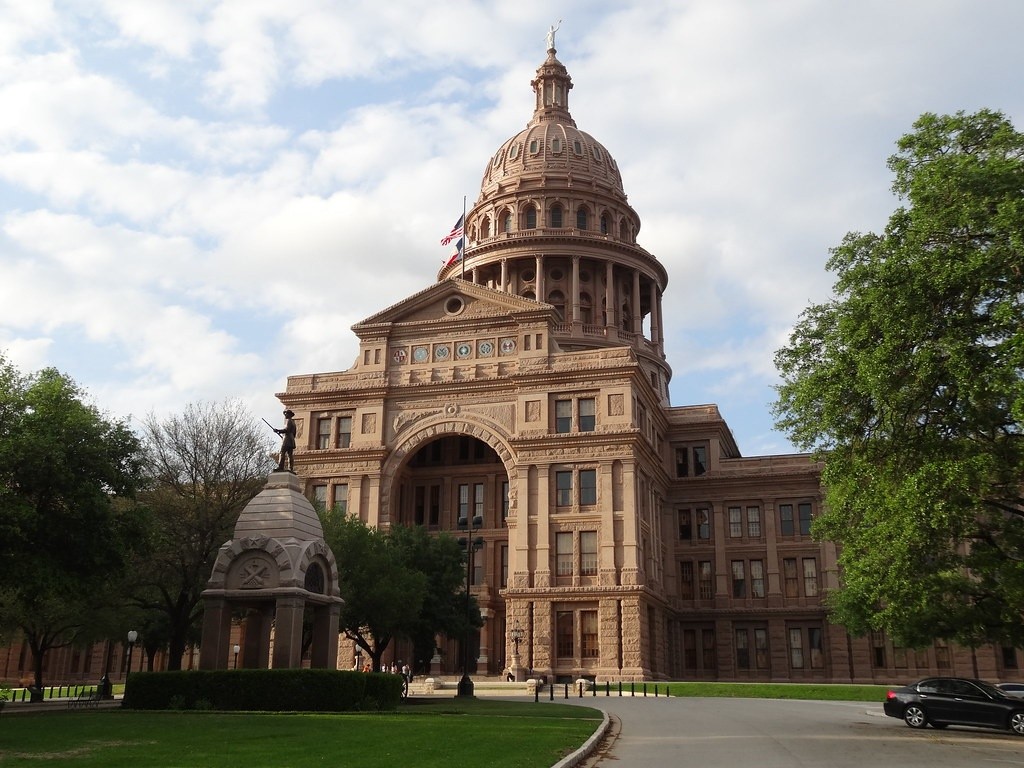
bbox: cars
[884,676,1024,736]
[994,683,1023,701]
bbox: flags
[441,237,463,268]
[440,212,463,247]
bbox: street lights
[125,631,137,684]
[356,644,361,671]
[234,644,240,668]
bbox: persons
[381,663,388,672]
[402,664,409,676]
[391,661,397,674]
